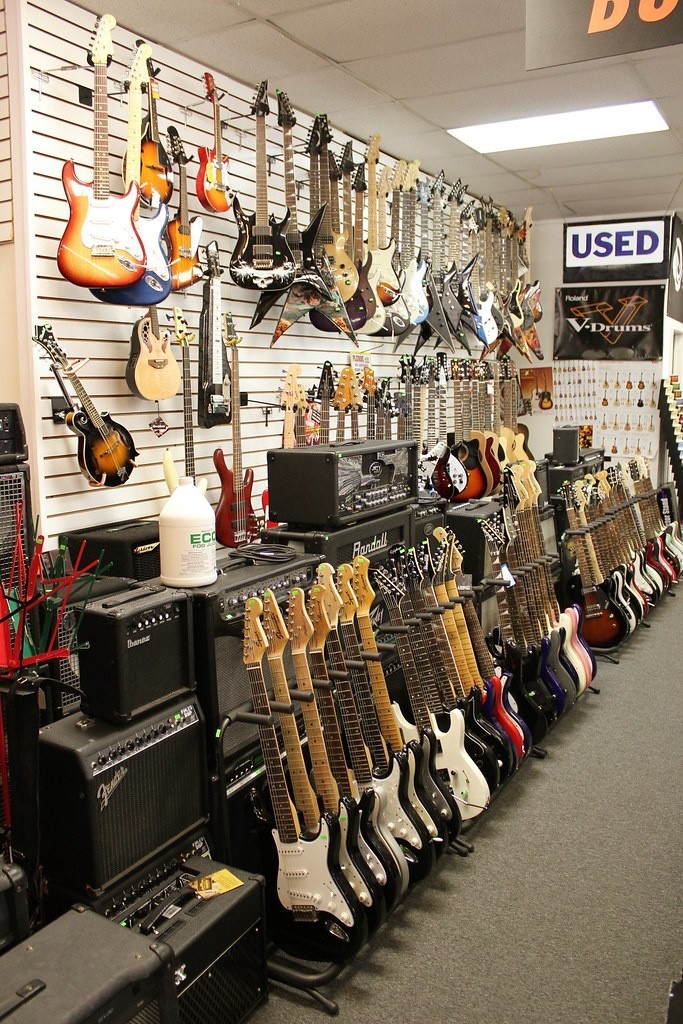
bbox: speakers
[547,426,605,551]
[0,435,501,1024]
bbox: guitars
[278,350,537,504]
[30,322,140,489]
[163,126,204,291]
[268,112,546,364]
[89,43,171,306]
[248,88,334,329]
[57,14,147,288]
[214,312,261,548]
[122,57,174,206]
[228,80,295,292]
[528,359,598,423]
[196,71,235,212]
[164,306,209,499]
[125,304,178,400]
[196,241,230,428]
[598,371,658,458]
[240,457,683,962]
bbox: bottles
[159,477,218,589]
